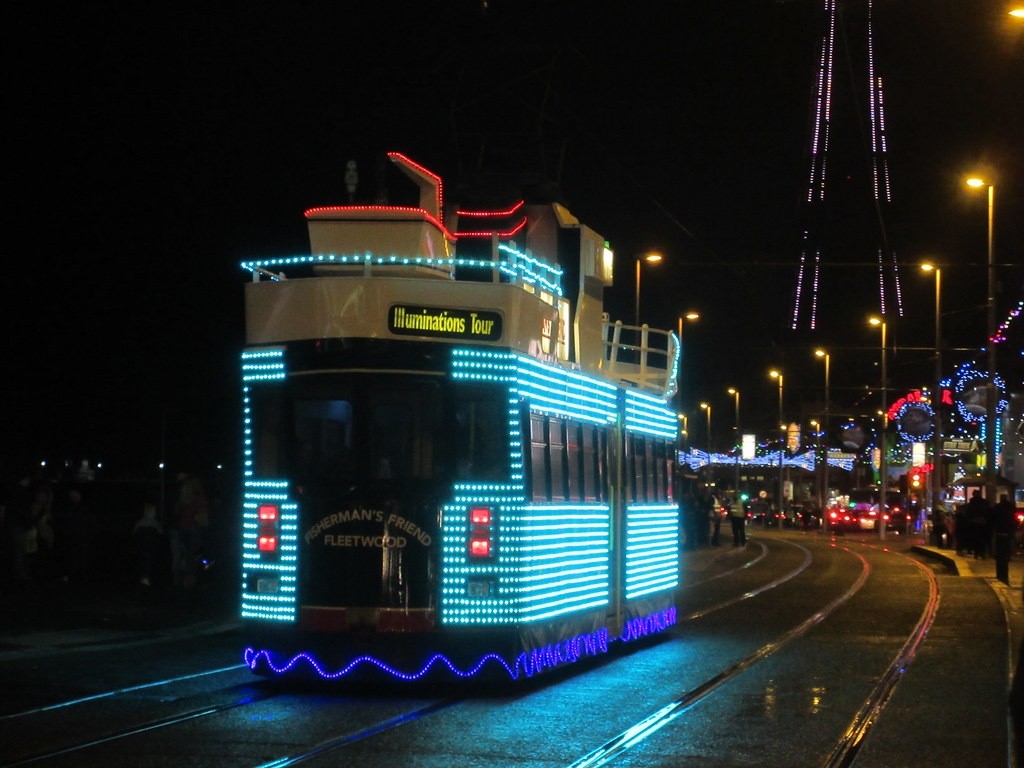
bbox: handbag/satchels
[193,496,208,527]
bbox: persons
[710,485,723,547]
[930,504,954,548]
[955,490,1018,563]
[0,458,212,618]
[727,491,748,547]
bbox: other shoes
[712,542,721,547]
[139,576,151,586]
[740,541,744,546]
[732,543,739,547]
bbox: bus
[238,146,688,683]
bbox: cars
[718,499,912,537]
[943,505,1024,556]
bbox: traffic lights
[912,475,921,489]
[741,494,749,502]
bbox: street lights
[769,368,784,528]
[677,312,700,411]
[919,262,940,543]
[866,315,886,541]
[634,250,662,361]
[699,402,711,493]
[680,412,689,462]
[964,172,994,506]
[728,389,740,503]
[816,348,828,532]
